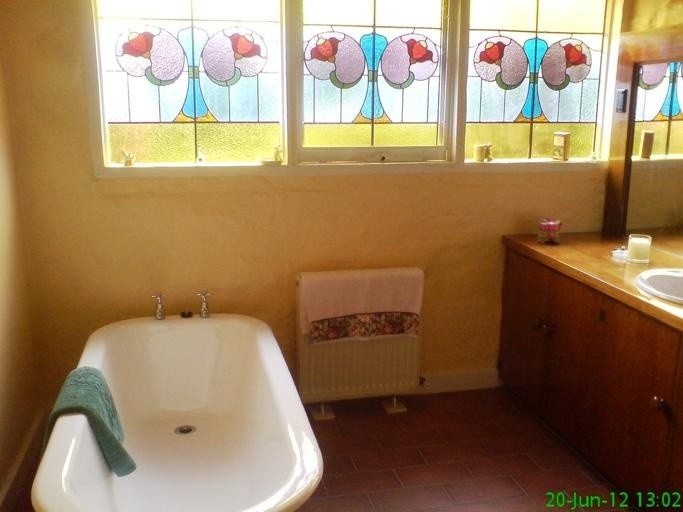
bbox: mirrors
[603,26,682,258]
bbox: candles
[629,237,650,260]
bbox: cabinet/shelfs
[498,248,683,511]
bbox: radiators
[296,268,432,421]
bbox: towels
[41,367,136,477]
[300,266,424,345]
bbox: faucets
[151,293,167,320]
[195,290,213,316]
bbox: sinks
[635,267,683,304]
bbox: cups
[627,233,652,263]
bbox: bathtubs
[32,312,324,512]
